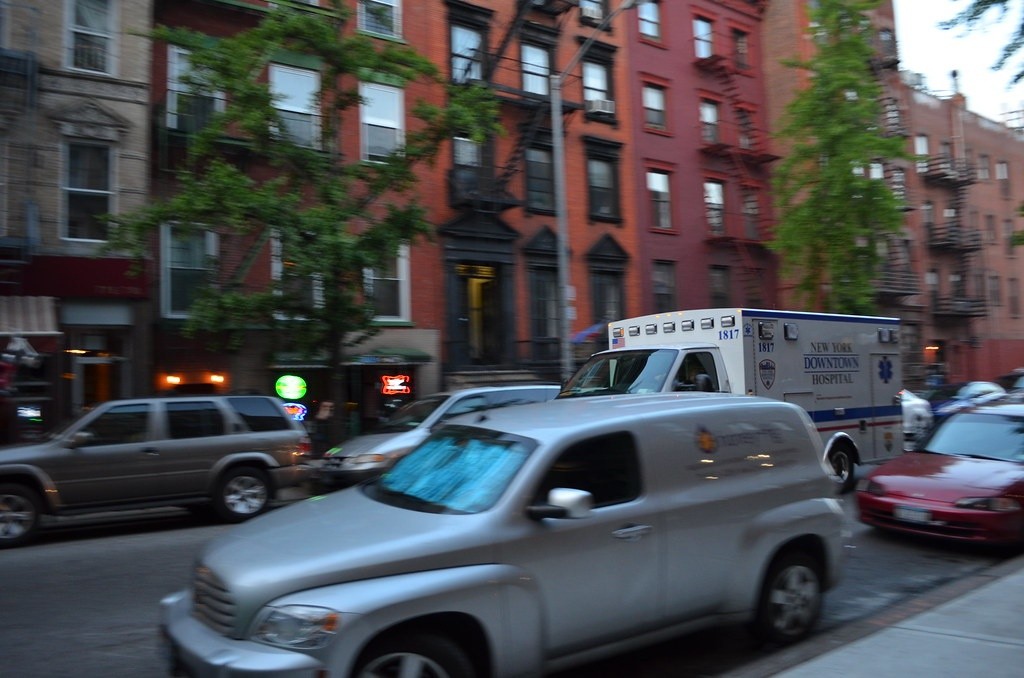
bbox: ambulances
[554,306,905,493]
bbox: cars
[1,380,62,437]
[901,369,1024,456]
[302,385,564,496]
[855,398,1024,547]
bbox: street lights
[547,0,644,383]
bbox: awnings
[246,348,437,370]
[0,295,65,337]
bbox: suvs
[154,390,844,677]
[0,394,315,548]
